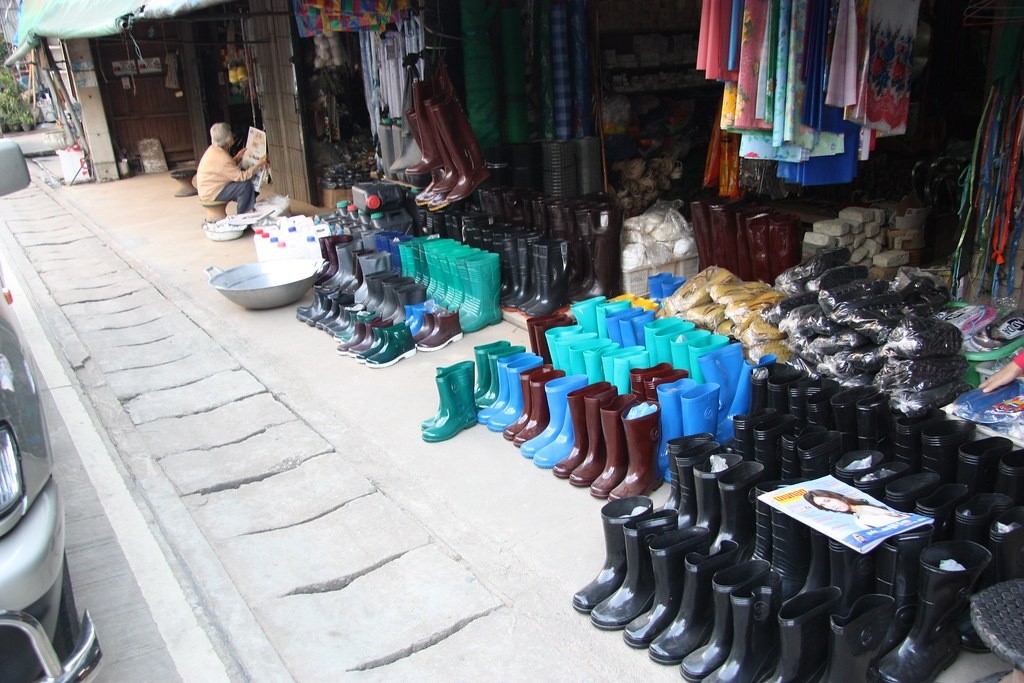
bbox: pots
[204,259,330,311]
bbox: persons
[196,122,270,215]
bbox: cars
[2,279,102,683]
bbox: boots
[419,270,1023,682]
[390,78,491,211]
[298,186,623,369]
